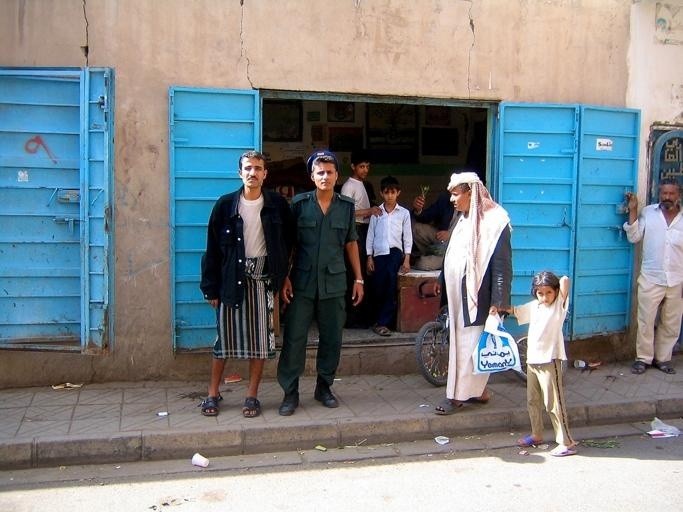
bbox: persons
[622,178,683,374]
[366,176,413,337]
[488,271,578,456]
[340,148,383,264]
[412,189,455,271]
[277,150,366,417]
[199,151,291,418]
[433,171,514,415]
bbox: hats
[304,149,339,179]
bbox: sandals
[199,395,219,416]
[652,358,675,375]
[372,322,393,337]
[629,360,648,375]
[242,397,260,419]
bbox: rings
[441,240,444,243]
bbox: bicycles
[413,279,570,386]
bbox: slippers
[432,399,464,416]
[515,435,544,447]
[548,444,577,457]
[461,396,488,404]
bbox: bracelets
[353,279,364,285]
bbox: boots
[277,376,300,416]
[312,373,339,409]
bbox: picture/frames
[327,102,355,122]
[263,99,303,142]
[366,103,419,163]
